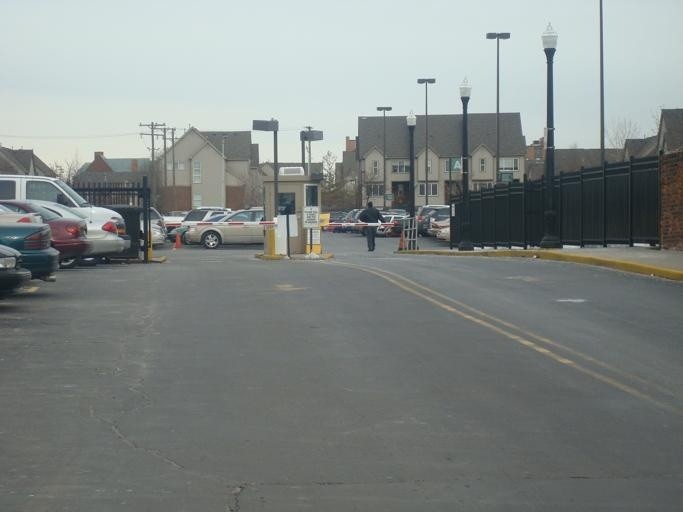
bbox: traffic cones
[396,233,407,251]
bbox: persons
[358,202,385,252]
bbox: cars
[136,173,267,258]
[0,244,33,291]
[0,199,93,259]
[325,203,451,243]
[0,221,60,284]
[0,203,43,224]
[3,199,119,268]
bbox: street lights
[219,134,228,206]
[538,21,558,176]
[404,109,418,250]
[299,129,323,170]
[415,78,435,204]
[485,32,511,182]
[458,73,473,192]
[251,117,278,219]
[375,106,393,206]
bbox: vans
[0,173,131,266]
